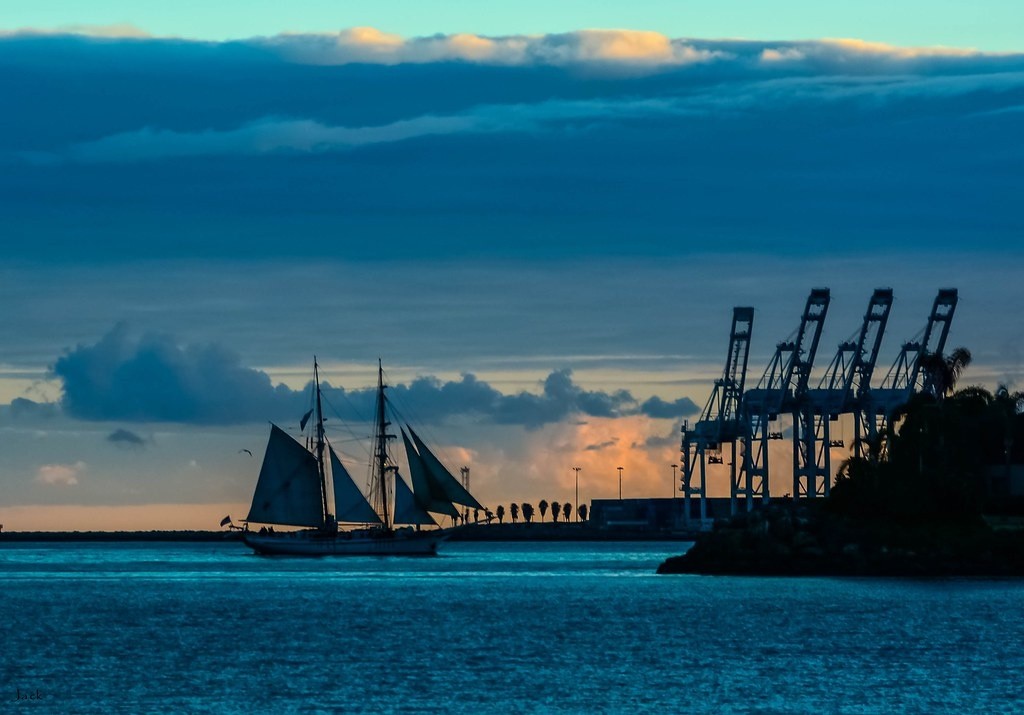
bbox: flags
[300,408,314,432]
[220,515,231,527]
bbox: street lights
[573,468,581,521]
[618,467,623,499]
[671,464,678,498]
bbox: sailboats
[220,354,497,555]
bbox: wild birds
[238,448,253,457]
